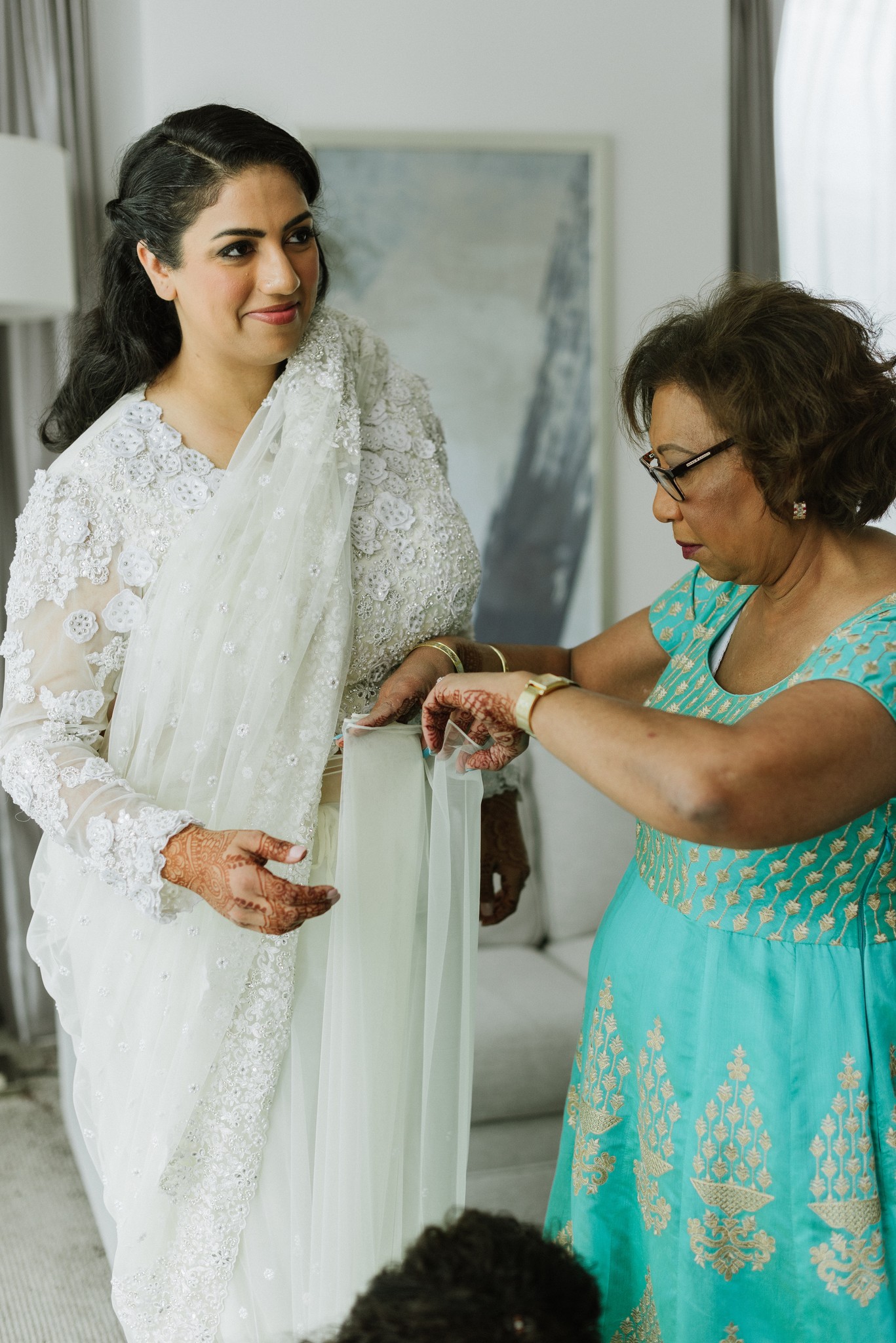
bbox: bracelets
[487,644,508,672]
[405,641,465,673]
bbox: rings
[436,677,445,685]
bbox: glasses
[638,434,739,502]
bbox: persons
[336,279,896,1341]
[2,106,532,1341]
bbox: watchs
[515,672,582,741]
